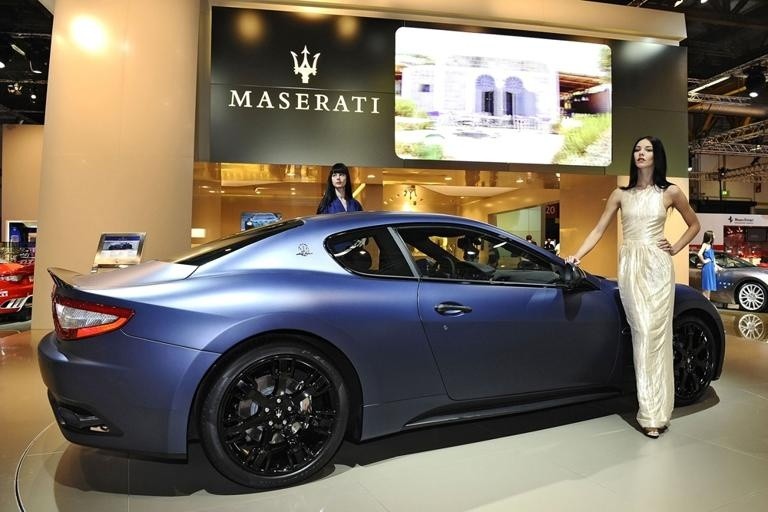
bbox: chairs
[347,236,414,276]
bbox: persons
[697,230,723,300]
[317,163,370,257]
[486,241,500,270]
[544,237,554,252]
[564,136,700,438]
[520,235,537,262]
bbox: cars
[689,250,768,312]
[37,211,725,488]
[716,307,767,341]
[0,258,34,315]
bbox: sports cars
[108,242,132,250]
[245,212,283,230]
